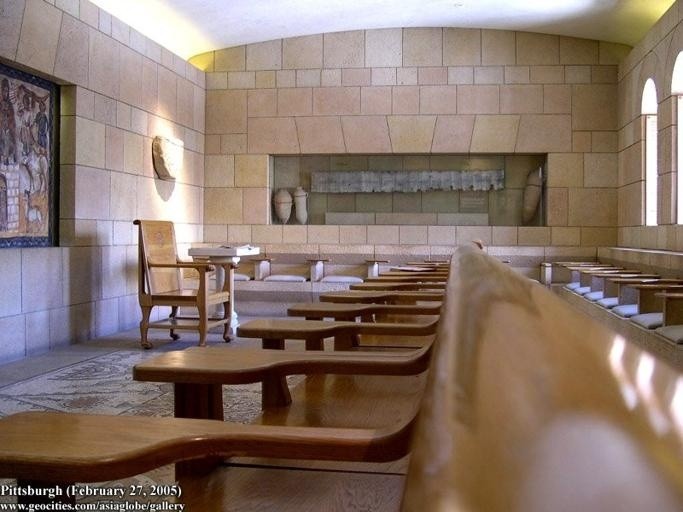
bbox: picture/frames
[0,66,60,248]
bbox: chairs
[134,316,618,383]
[0,340,683,512]
[351,246,542,299]
[237,302,604,352]
[286,283,575,329]
[134,219,240,349]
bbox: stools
[565,264,683,345]
[315,253,374,298]
[187,258,257,318]
[257,260,314,313]
